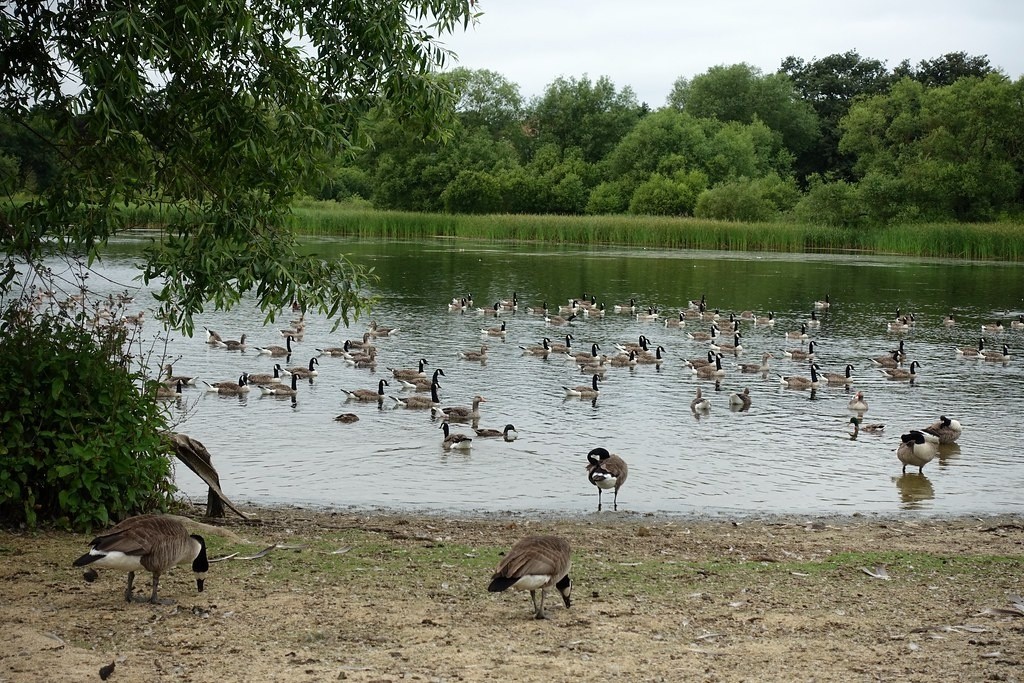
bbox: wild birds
[848,417,887,432]
[315,320,400,366]
[897,415,962,474]
[142,364,198,402]
[72,514,209,604]
[202,375,249,392]
[243,364,302,394]
[332,358,487,449]
[252,316,304,355]
[488,536,572,619]
[202,325,248,348]
[448,291,921,414]
[585,448,628,511]
[281,357,319,376]
[942,309,1024,359]
[475,423,518,436]
